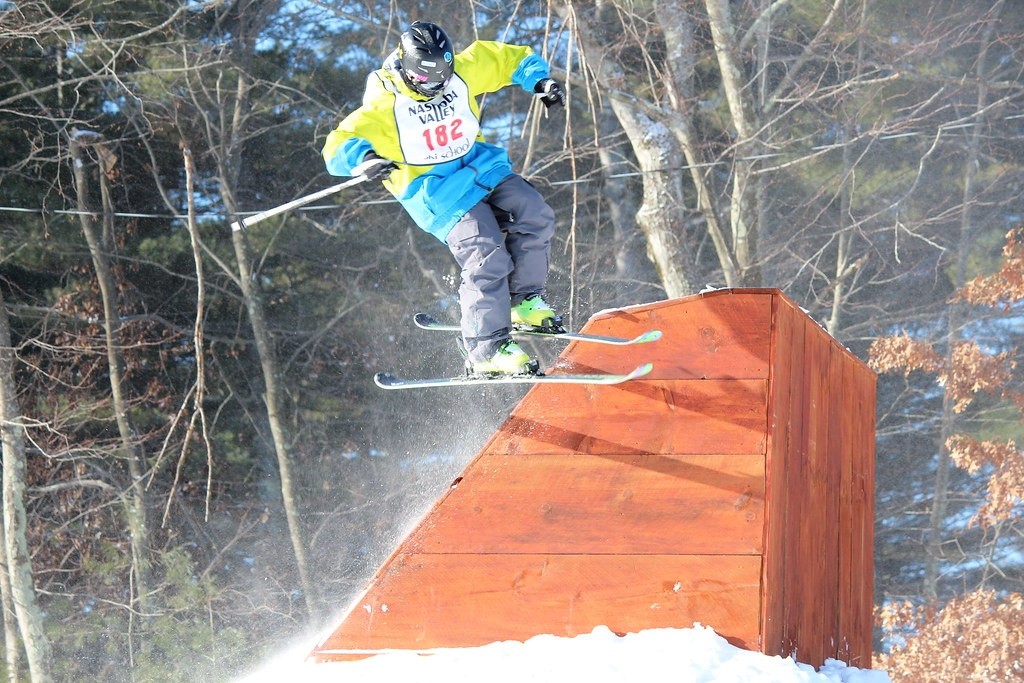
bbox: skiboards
[374,311,664,388]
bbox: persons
[321,22,567,377]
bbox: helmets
[396,19,455,98]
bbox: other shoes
[466,339,540,377]
[510,293,562,334]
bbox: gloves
[364,153,399,186]
[534,78,567,108]
